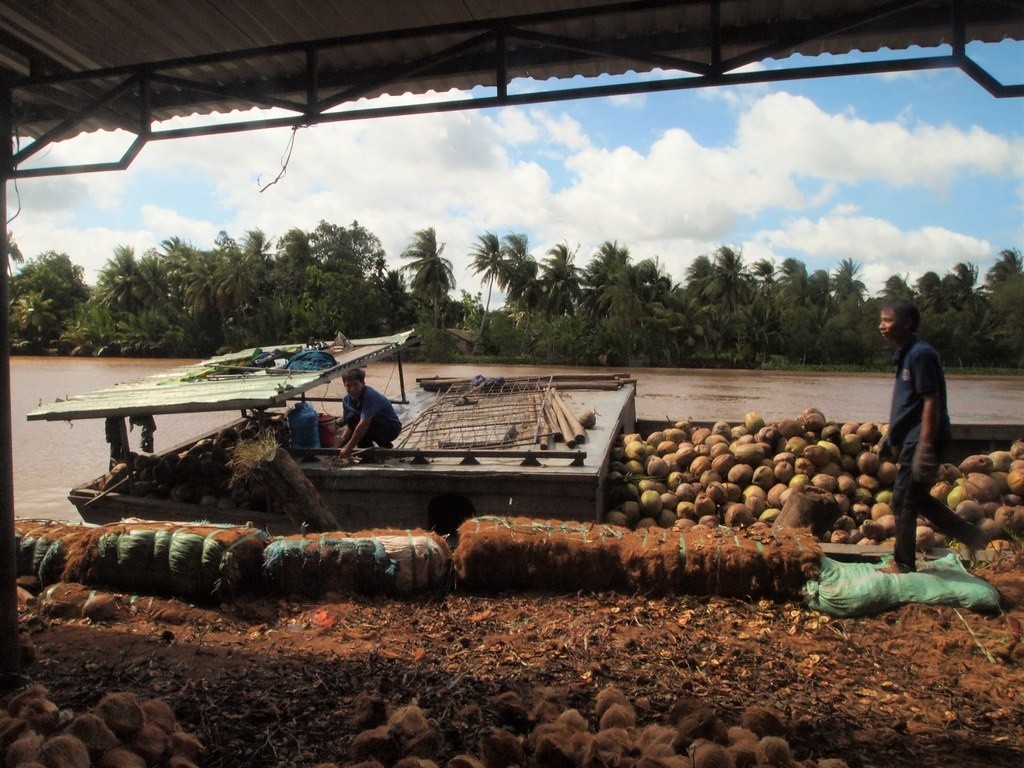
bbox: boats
[25,328,1024,539]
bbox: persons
[337,367,403,459]
[875,299,988,573]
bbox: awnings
[0,0,1024,183]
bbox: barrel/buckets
[319,413,337,448]
[287,402,321,447]
